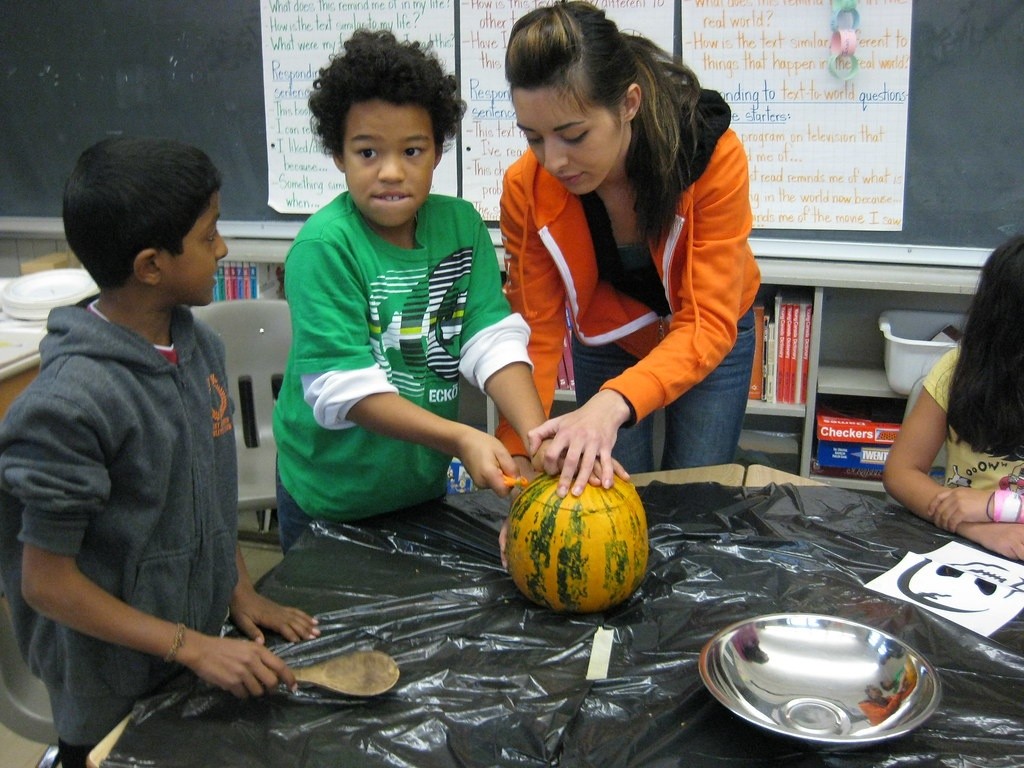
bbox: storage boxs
[877,310,969,396]
[21,252,82,276]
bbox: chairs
[0,597,61,768]
[189,299,291,530]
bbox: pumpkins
[506,470,647,608]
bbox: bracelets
[989,476,1021,526]
[162,617,187,669]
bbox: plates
[697,613,945,746]
[0,269,101,321]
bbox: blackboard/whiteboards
[0,0,1024,298]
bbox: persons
[878,237,1024,569]
[0,134,319,767]
[272,0,761,571]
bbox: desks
[0,277,100,424]
[88,465,1024,768]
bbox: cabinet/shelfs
[0,217,996,495]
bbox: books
[818,403,903,477]
[214,260,260,302]
[748,291,812,407]
